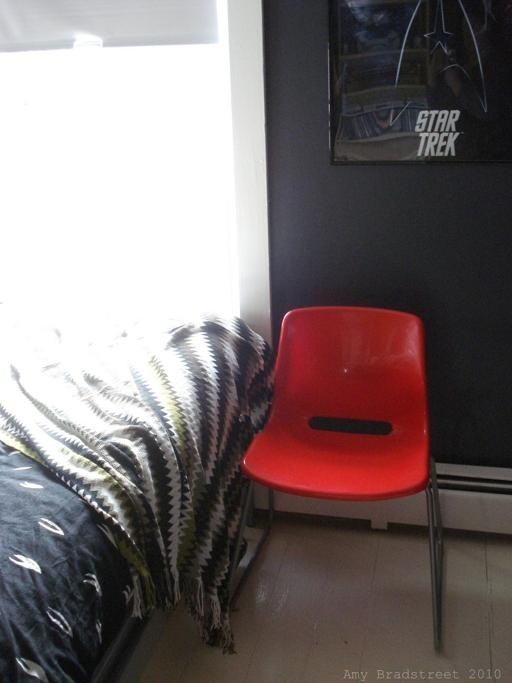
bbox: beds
[1,311,274,683]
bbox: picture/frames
[326,0,512,164]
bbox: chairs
[222,305,444,651]
